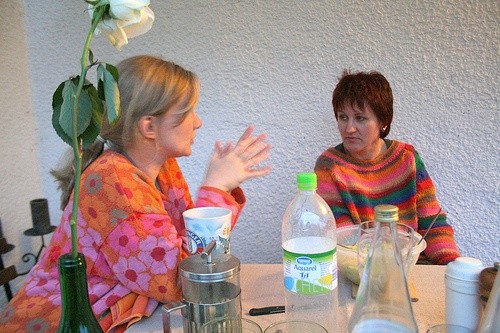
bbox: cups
[200,317,262,333]
[182,207,232,255]
[358,221,414,281]
[444,257,484,333]
[264,320,329,333]
[426,324,473,333]
[162,253,242,333]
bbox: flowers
[52,0,155,308]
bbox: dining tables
[127,263,499,333]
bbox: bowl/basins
[336,225,427,285]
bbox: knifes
[249,306,317,316]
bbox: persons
[0,55,273,333]
[313,67,461,264]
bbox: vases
[55,253,107,333]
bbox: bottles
[281,173,339,333]
[349,204,418,333]
[56,253,104,333]
